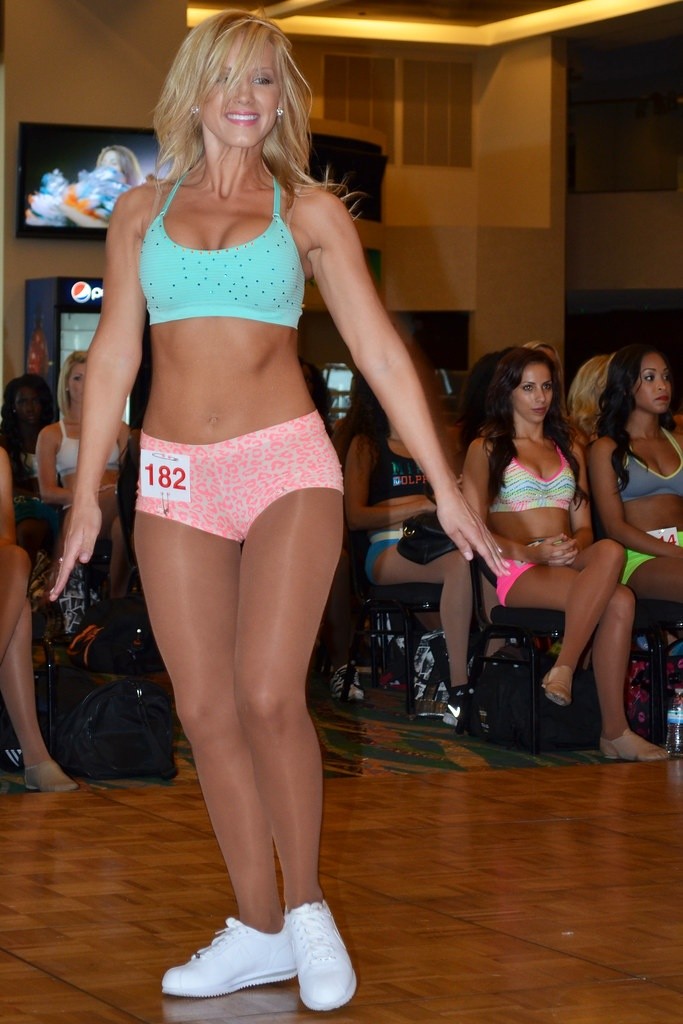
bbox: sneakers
[162,918,298,997]
[329,665,364,702]
[284,899,357,1011]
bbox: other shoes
[443,684,472,727]
[24,760,79,793]
[600,728,669,761]
[542,666,573,705]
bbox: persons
[0,351,133,790]
[95,143,144,188]
[48,10,512,1011]
[298,344,683,762]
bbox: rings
[58,558,63,564]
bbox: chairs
[456,555,682,754]
[32,561,111,755]
[340,527,444,720]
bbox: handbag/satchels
[372,627,488,702]
[470,644,603,751]
[28,547,167,675]
[397,513,459,565]
[0,664,180,781]
